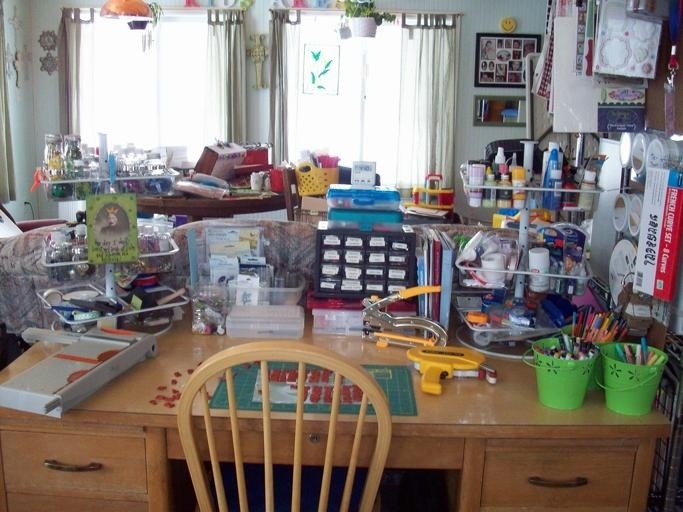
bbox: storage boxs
[193,139,463,334]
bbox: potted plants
[344,0,396,39]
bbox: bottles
[41,133,83,181]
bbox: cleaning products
[463,142,566,211]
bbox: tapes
[73,310,102,323]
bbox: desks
[0,314,673,512]
[139,190,298,223]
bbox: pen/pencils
[298,152,340,171]
[533,304,665,365]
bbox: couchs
[170,219,539,294]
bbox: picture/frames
[473,33,542,88]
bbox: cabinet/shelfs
[455,140,606,345]
[36,164,191,336]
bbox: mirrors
[473,94,527,127]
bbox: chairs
[176,343,391,512]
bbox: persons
[98,212,122,234]
[479,40,536,83]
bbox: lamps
[100,0,154,21]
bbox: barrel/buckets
[595,342,669,416]
[521,336,601,410]
[559,325,612,391]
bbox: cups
[528,247,550,293]
[250,174,263,191]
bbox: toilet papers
[482,252,505,283]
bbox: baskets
[295,160,340,197]
